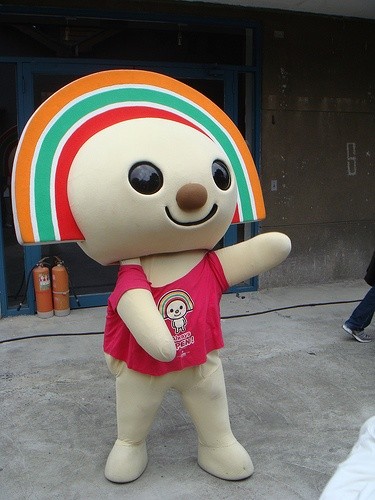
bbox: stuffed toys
[13,67,293,483]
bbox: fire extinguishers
[32,263,54,319]
[51,256,71,317]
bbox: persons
[342,255,374,342]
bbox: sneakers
[342,323,372,343]
